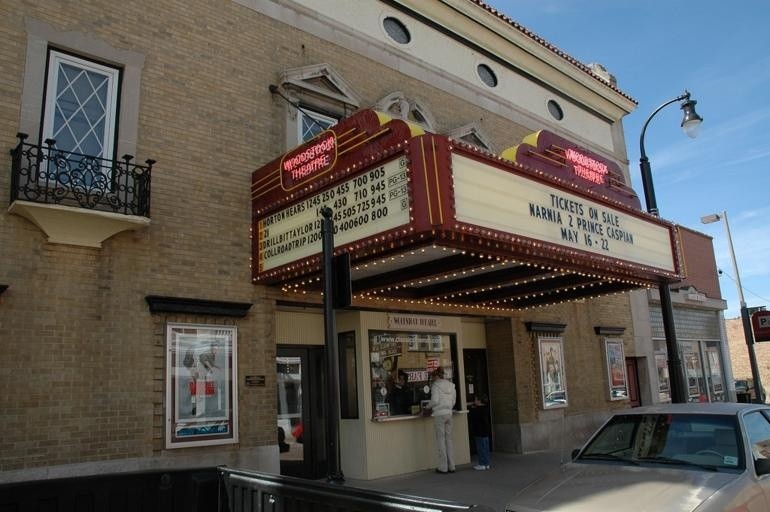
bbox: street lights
[638,90,705,405]
[701,211,762,404]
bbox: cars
[504,400,769,512]
[734,377,766,404]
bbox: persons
[548,347,555,363]
[470,392,491,471]
[200,342,224,373]
[390,370,413,414]
[425,367,457,474]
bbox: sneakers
[435,467,455,474]
[473,464,491,470]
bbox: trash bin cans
[736,393,752,403]
